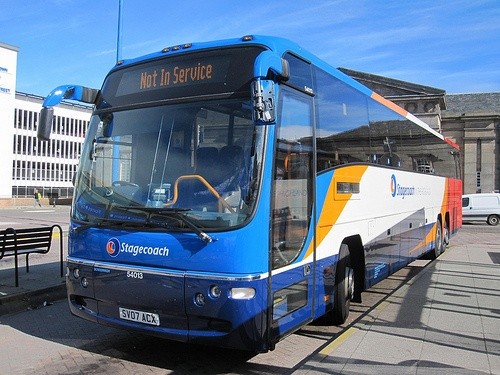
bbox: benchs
[0,225,64,288]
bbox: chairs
[152,145,252,200]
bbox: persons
[33,190,42,208]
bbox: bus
[37,36,464,352]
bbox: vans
[461,192,500,227]
